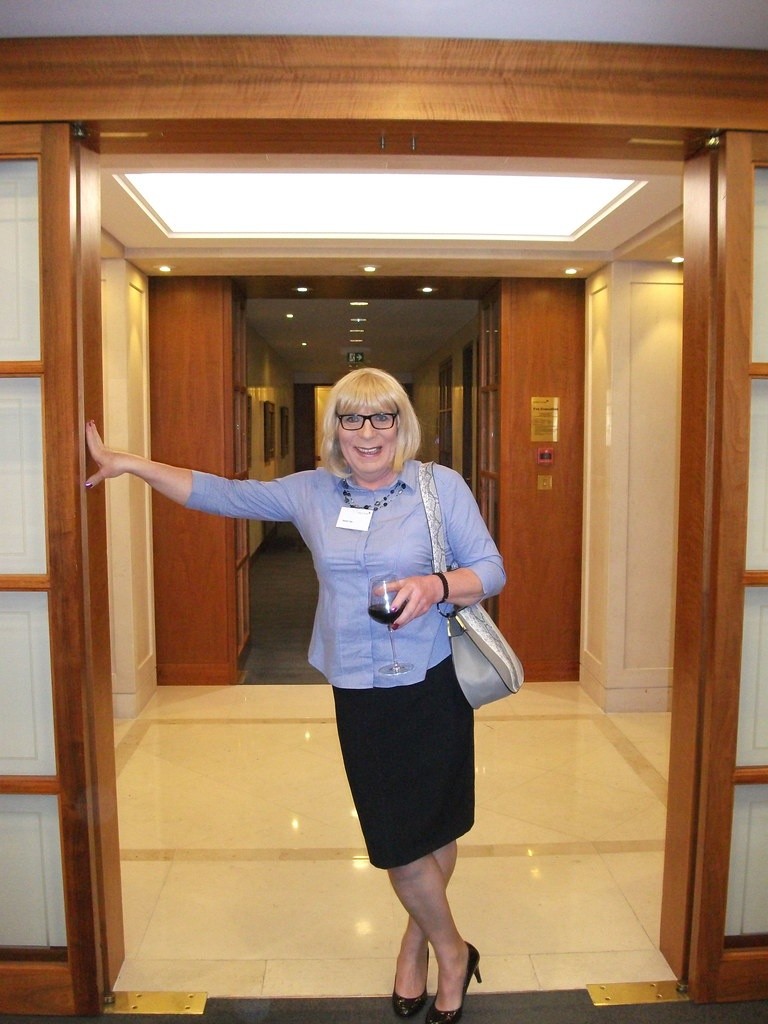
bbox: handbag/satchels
[443,600,525,712]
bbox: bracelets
[432,572,449,604]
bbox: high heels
[391,945,430,1017]
[425,939,482,1023]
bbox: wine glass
[369,574,416,676]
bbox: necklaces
[340,470,406,512]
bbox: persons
[77,365,505,1024]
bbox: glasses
[335,408,400,431]
